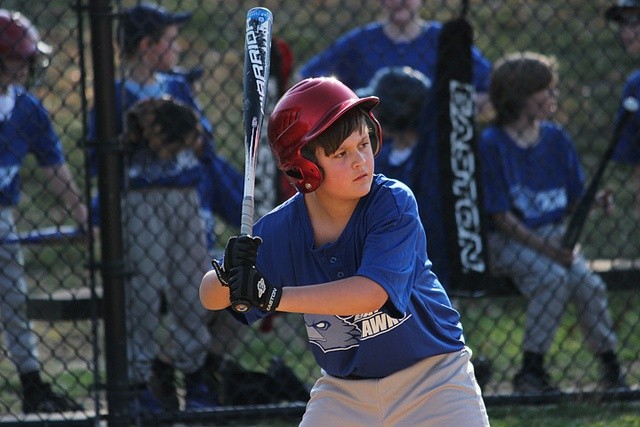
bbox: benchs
[24,286,169,322]
[487,257,640,299]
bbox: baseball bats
[229,7,273,312]
[1,226,87,245]
[558,94,640,248]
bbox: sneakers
[148,374,178,411]
[20,384,82,413]
[133,390,165,415]
[513,370,559,395]
[185,383,222,408]
[600,366,627,387]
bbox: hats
[117,6,192,46]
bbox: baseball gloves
[119,94,205,164]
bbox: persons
[86,2,216,419]
[1,10,88,413]
[161,62,246,383]
[606,0,639,167]
[351,64,494,394]
[474,48,632,395]
[197,75,490,427]
[295,0,496,118]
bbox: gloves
[226,267,282,313]
[212,235,263,285]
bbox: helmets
[1,10,52,61]
[351,66,432,130]
[267,76,382,193]
